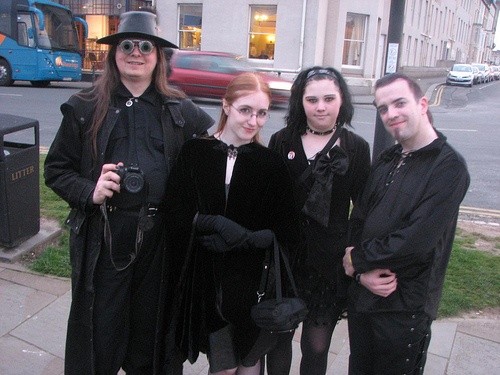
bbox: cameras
[110,165,146,193]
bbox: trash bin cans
[0,113,41,248]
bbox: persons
[344,73,470,375]
[43,11,215,375]
[163,72,298,375]
[268,66,372,375]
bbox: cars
[446,63,475,87]
[167,49,296,104]
[471,63,500,84]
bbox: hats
[96,11,179,49]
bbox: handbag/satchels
[251,233,307,336]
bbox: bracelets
[352,271,362,285]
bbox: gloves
[197,215,256,256]
[197,230,273,253]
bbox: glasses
[117,39,153,54]
[229,103,270,122]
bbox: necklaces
[306,126,336,136]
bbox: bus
[0,0,88,88]
